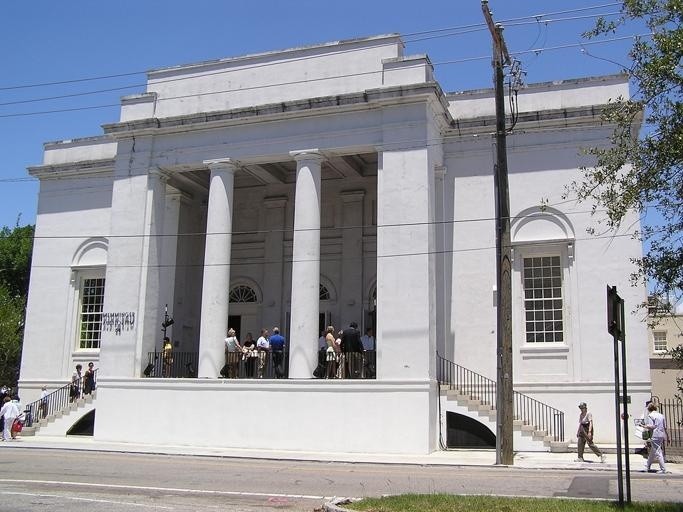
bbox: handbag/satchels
[642,431,652,440]
[12,420,22,432]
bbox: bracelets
[587,430,591,433]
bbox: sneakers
[574,457,584,462]
[656,470,666,474]
[639,468,649,472]
[601,454,606,463]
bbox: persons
[311,321,375,379]
[224,328,243,377]
[267,326,285,378]
[161,337,173,378]
[573,401,606,463]
[255,328,269,378]
[81,362,95,395]
[241,332,256,378]
[0,391,23,441]
[68,364,82,404]
[39,384,48,419]
[638,403,671,474]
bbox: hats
[578,402,587,409]
